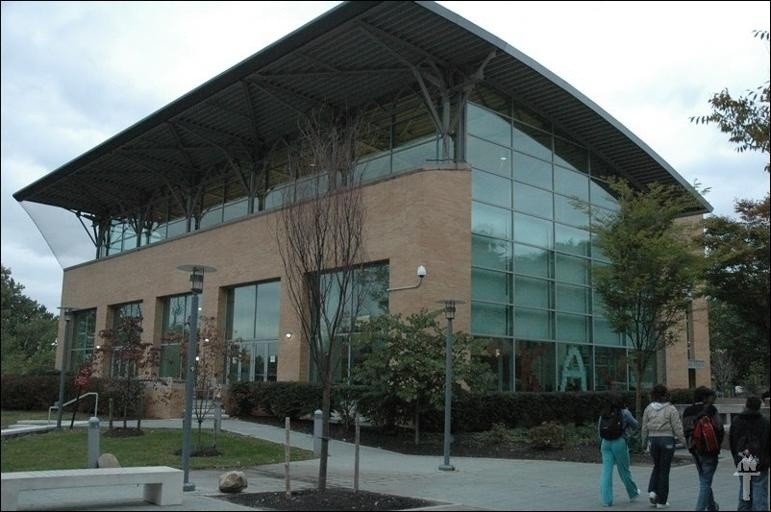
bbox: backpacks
[688,403,721,456]
[735,429,761,472]
[599,404,629,440]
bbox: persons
[641,383,690,509]
[728,393,771,511]
[598,390,643,506]
[681,384,725,511]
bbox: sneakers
[629,488,641,502]
[647,492,670,509]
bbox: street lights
[53,305,81,429]
[437,296,467,471]
[174,261,218,491]
